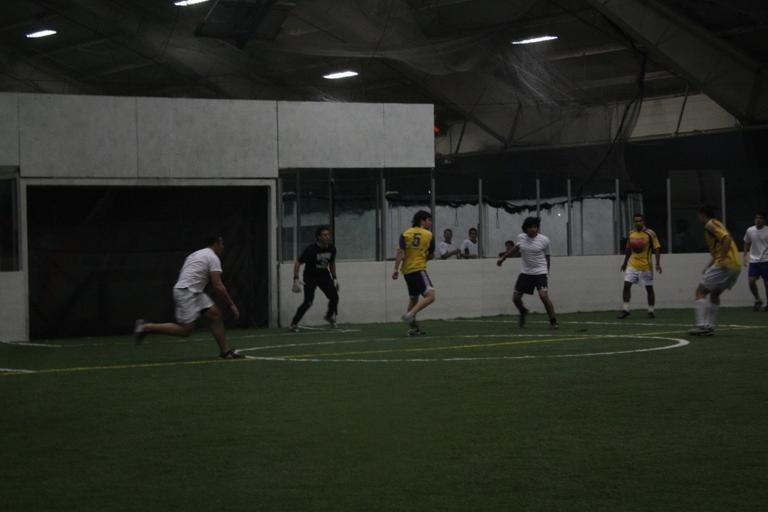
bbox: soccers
[631,238,645,253]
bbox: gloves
[291,279,306,294]
[333,279,339,291]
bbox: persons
[461,228,480,258]
[391,210,436,337]
[289,226,341,334]
[742,212,768,312]
[438,228,461,260]
[134,232,244,359]
[686,205,743,335]
[617,213,663,319]
[498,217,559,330]
[499,240,521,257]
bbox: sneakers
[324,316,337,328]
[752,300,762,312]
[134,318,145,345]
[703,329,714,336]
[687,325,712,333]
[616,310,631,320]
[401,312,416,327]
[761,304,768,312]
[648,312,654,318]
[288,321,299,332]
[519,308,528,327]
[218,350,246,360]
[553,323,559,329]
[408,329,426,337]
